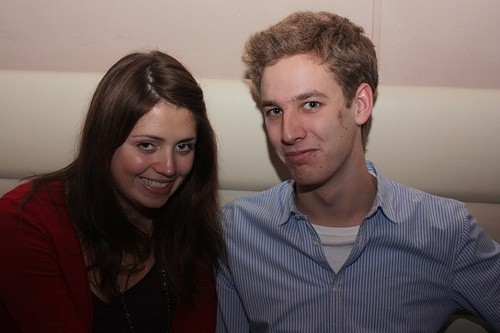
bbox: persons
[0,49,219,333]
[217,11,500,333]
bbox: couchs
[0,69,500,333]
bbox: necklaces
[116,264,172,333]
